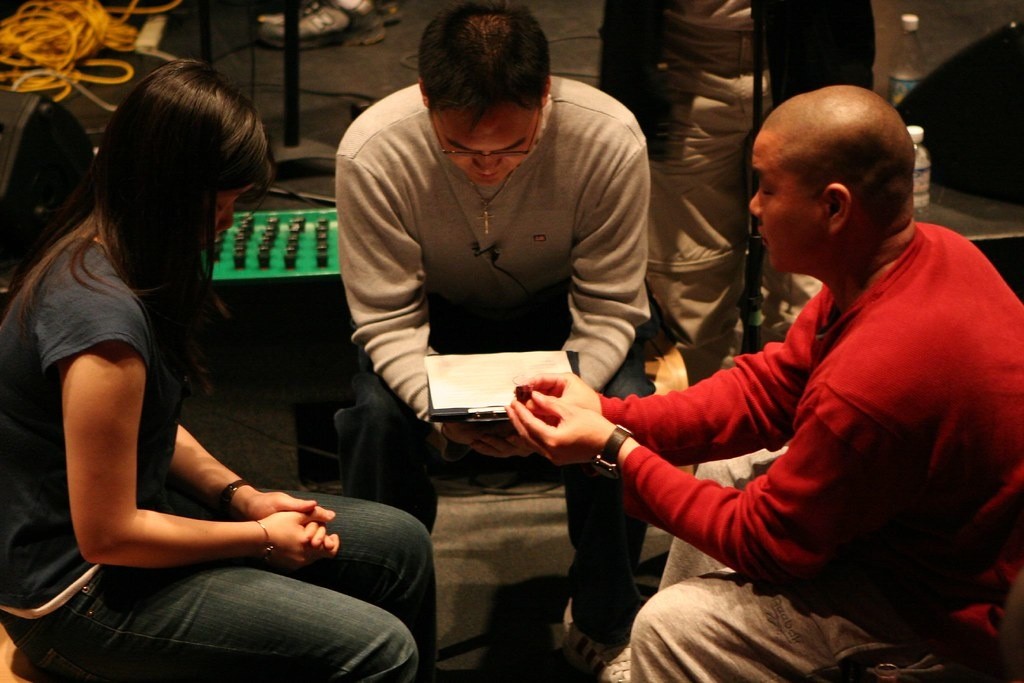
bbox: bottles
[907,126,931,208]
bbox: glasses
[429,105,541,157]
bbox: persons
[505,85,1024,683]
[254,0,402,52]
[328,0,652,683]
[596,0,877,390]
[0,59,438,683]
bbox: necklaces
[472,170,513,235]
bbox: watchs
[591,423,633,482]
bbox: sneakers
[563,623,646,683]
[257,0,401,48]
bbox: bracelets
[220,479,254,506]
[255,519,275,561]
